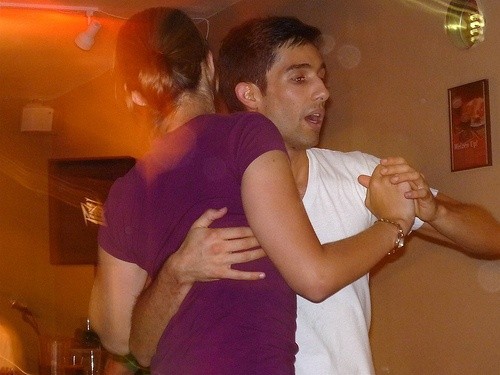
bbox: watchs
[375,218,405,256]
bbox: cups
[38,337,67,375]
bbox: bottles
[69,319,102,375]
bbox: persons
[89,6,414,375]
[128,15,500,375]
